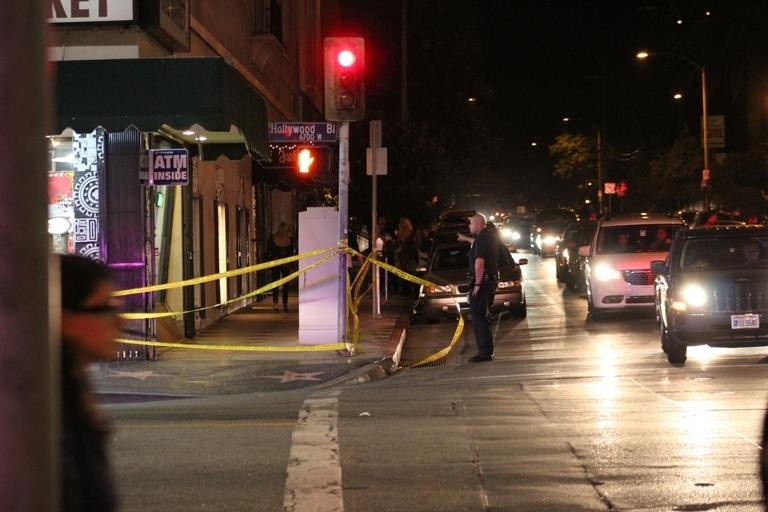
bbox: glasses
[66,299,121,318]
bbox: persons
[739,240,768,266]
[57,252,127,511]
[611,231,635,251]
[646,226,673,249]
[342,215,437,298]
[268,221,296,312]
[456,213,499,362]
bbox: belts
[483,275,497,280]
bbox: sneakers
[467,353,495,363]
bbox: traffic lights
[297,147,314,173]
[334,42,360,111]
